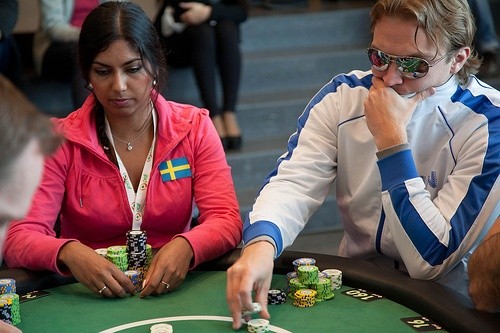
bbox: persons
[153,0,249,154]
[0,0,129,110]
[2,0,242,299]
[225,0,500,329]
[0,73,62,333]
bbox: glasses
[366,46,456,79]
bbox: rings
[160,279,170,289]
[99,285,106,293]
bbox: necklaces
[112,119,153,151]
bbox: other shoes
[227,135,242,149]
[220,136,227,152]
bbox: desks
[0,251,500,333]
